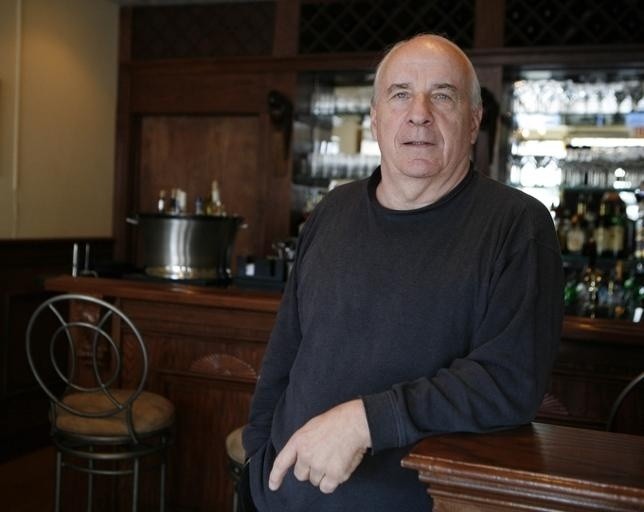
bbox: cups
[303,93,380,179]
[509,80,644,191]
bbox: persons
[236,33,565,511]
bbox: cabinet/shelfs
[289,68,644,327]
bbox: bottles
[155,178,228,217]
[559,196,635,319]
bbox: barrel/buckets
[138,212,245,278]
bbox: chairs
[27,294,176,511]
